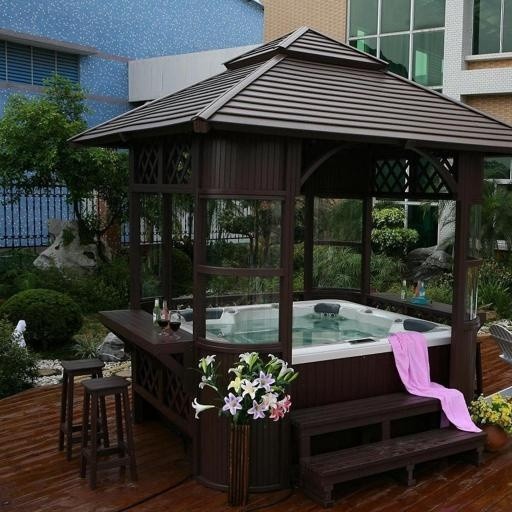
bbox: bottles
[153,298,162,328]
[400,279,408,302]
[415,280,426,297]
[160,300,169,322]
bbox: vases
[480,423,510,451]
[229,419,248,512]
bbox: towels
[386,330,484,434]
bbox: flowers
[190,348,301,424]
[466,392,511,427]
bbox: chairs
[488,323,511,364]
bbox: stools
[53,360,110,463]
[81,374,139,490]
[472,337,484,397]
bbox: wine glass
[168,311,181,339]
[157,318,169,337]
